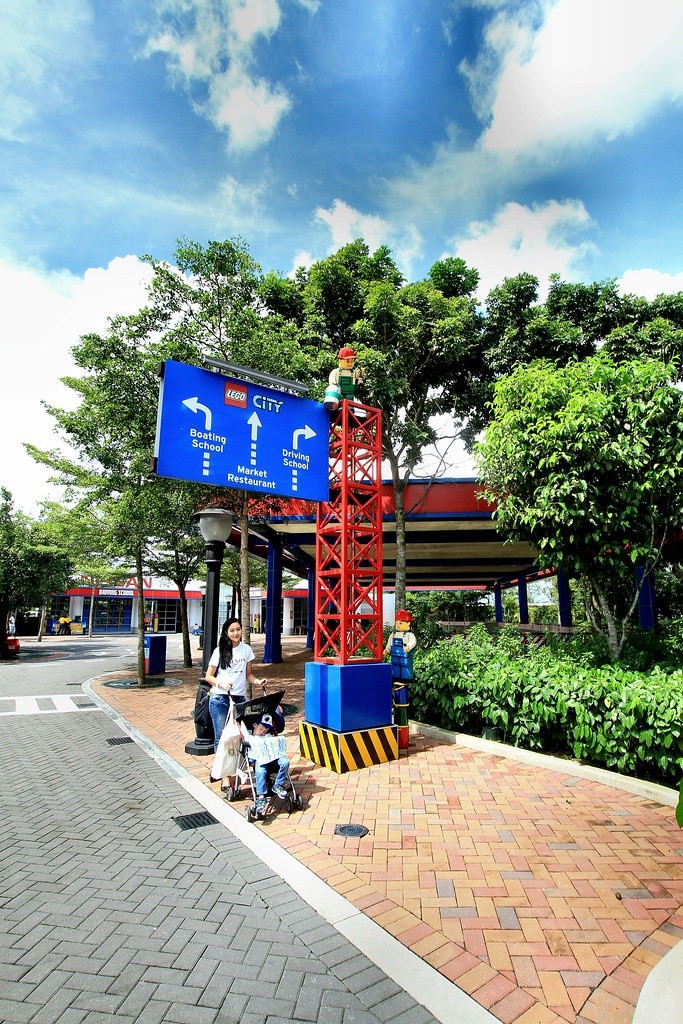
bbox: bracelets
[218,683,221,687]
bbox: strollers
[209,680,303,823]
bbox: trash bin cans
[144,635,166,675]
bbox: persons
[248,713,290,815]
[383,609,417,682]
[191,623,200,636]
[323,347,364,415]
[205,619,268,790]
[8,613,16,636]
[56,614,71,635]
[254,619,258,633]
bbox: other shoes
[221,782,230,793]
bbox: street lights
[225,594,232,620]
[197,585,206,651]
[183,507,241,758]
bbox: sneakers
[254,798,268,814]
[271,784,288,799]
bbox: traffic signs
[158,362,332,502]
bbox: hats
[256,712,274,732]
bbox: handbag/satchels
[211,701,247,780]
[194,688,213,729]
